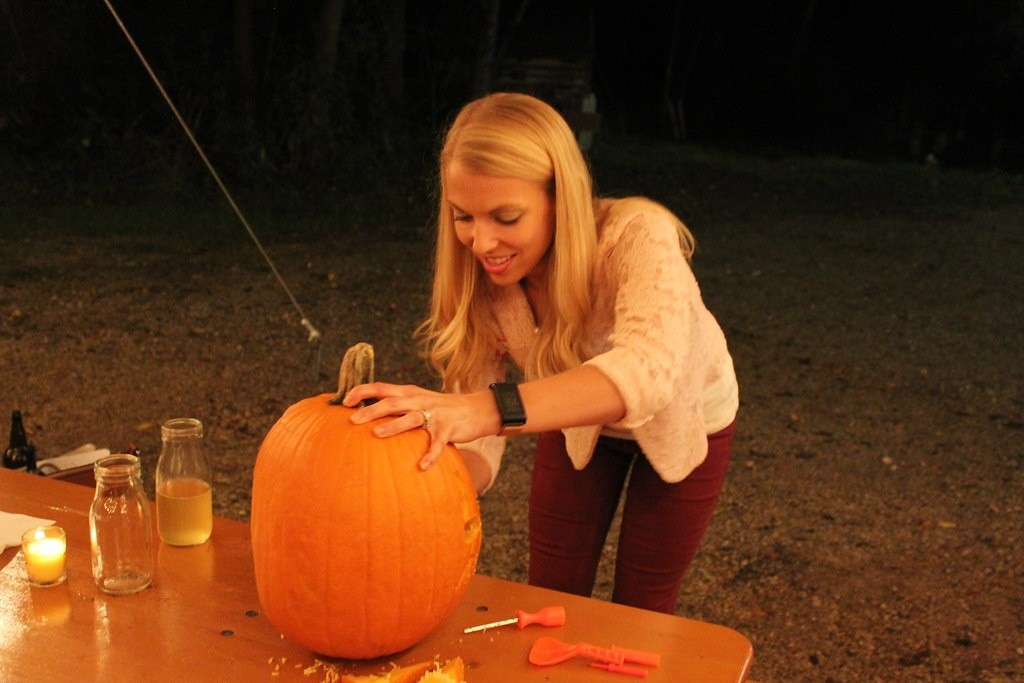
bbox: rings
[417,407,431,427]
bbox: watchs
[487,381,527,436]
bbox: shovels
[528,636,625,667]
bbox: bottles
[89,453,157,596]
[155,416,213,546]
[3,409,37,477]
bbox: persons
[344,91,739,615]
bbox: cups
[20,526,67,588]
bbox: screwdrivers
[590,662,648,678]
[463,605,565,634]
